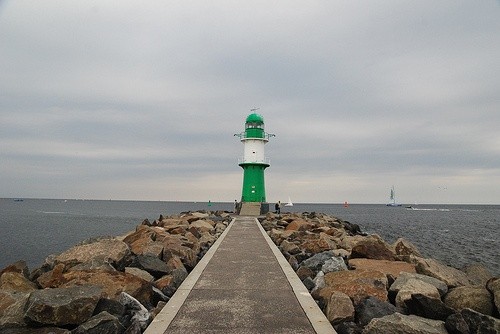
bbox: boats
[405,207,412,210]
[14,199,23,202]
[208,200,212,206]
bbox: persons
[274,201,281,214]
[233,200,238,214]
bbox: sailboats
[284,195,293,206]
[386,187,401,208]
